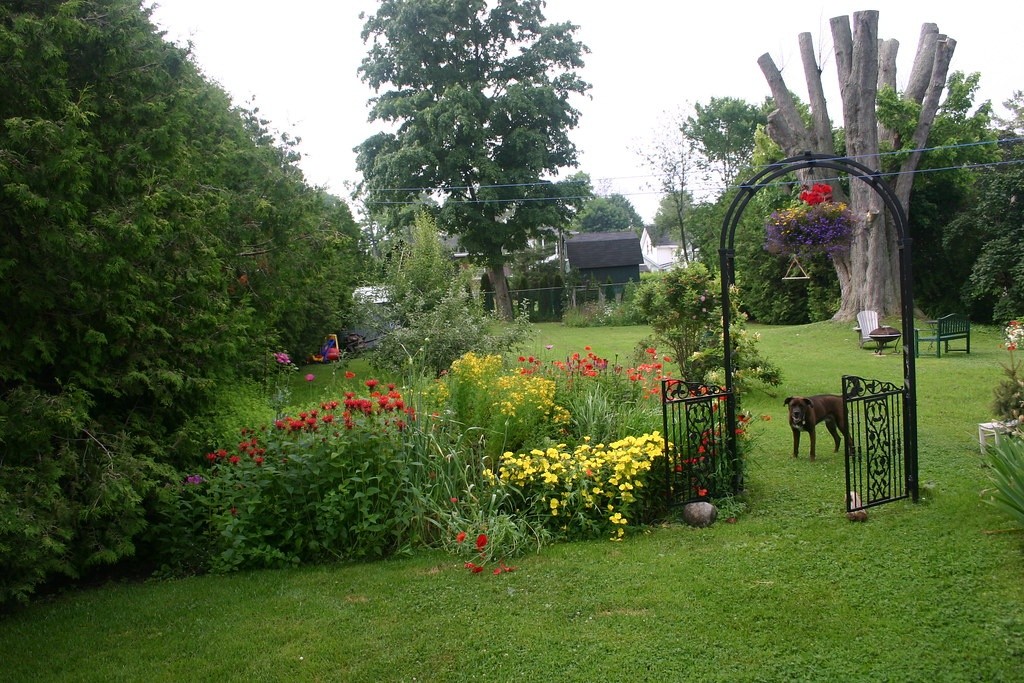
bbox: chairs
[853,310,882,348]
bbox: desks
[926,319,950,350]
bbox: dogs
[782,375,861,462]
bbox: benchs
[914,314,971,358]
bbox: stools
[978,421,1014,454]
